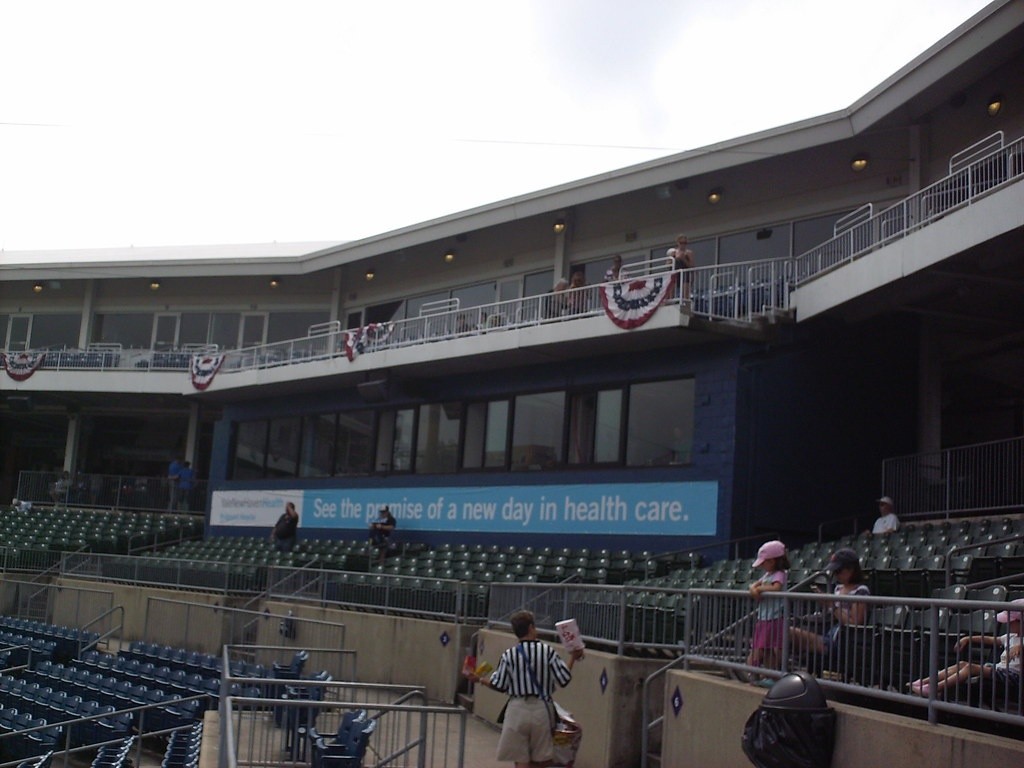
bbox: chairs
[1,344,120,368]
[670,276,793,319]
[135,348,205,369]
[0,504,1024,767]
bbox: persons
[478,609,575,768]
[905,598,1024,701]
[788,548,871,660]
[168,458,196,514]
[545,253,625,323]
[270,502,299,552]
[667,234,695,305]
[745,539,790,688]
[861,496,900,536]
[456,311,502,339]
[369,505,397,547]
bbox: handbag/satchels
[552,699,582,768]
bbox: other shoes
[906,678,923,689]
[911,683,941,700]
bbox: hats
[823,548,860,571]
[378,505,389,513]
[667,248,677,256]
[753,541,786,567]
[992,598,1024,624]
[876,496,893,505]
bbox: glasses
[679,241,688,245]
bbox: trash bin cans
[552,702,583,768]
[759,671,835,768]
[322,580,336,603]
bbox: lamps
[552,219,565,234]
[986,93,1002,118]
[850,154,868,171]
[365,268,377,279]
[444,249,456,261]
[706,187,723,205]
